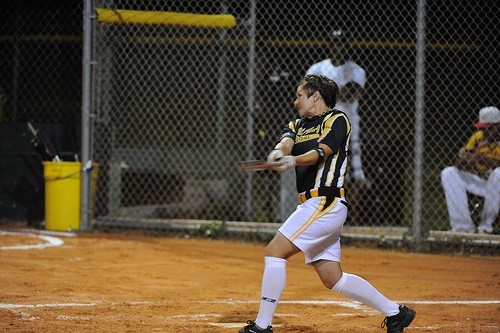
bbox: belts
[298,187,344,203]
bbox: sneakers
[238,320,273,333]
[380,304,416,333]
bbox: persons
[238,74,417,333]
[304,34,365,183]
[441,106,500,235]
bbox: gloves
[267,143,296,174]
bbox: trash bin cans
[43,160,99,234]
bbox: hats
[327,29,349,46]
[474,106,500,127]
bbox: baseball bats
[238,160,280,173]
